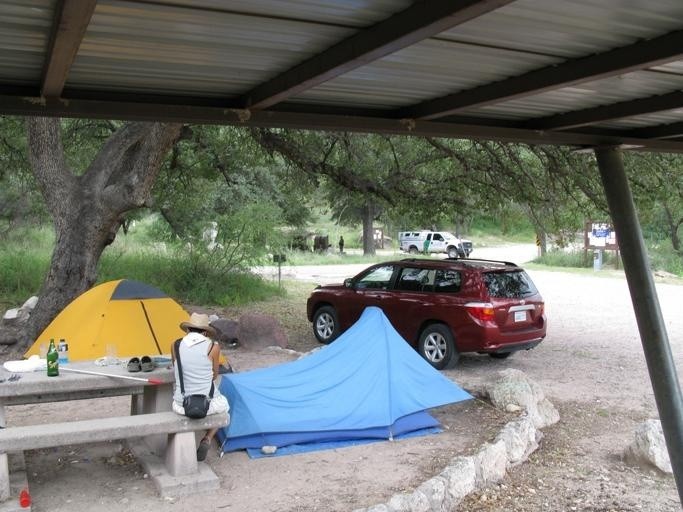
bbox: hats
[307,259,546,370]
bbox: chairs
[196,436,211,461]
[141,355,154,372]
[127,357,141,372]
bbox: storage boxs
[0,411,230,502]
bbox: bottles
[179,312,213,333]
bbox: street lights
[182,394,211,419]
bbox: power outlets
[400,231,473,258]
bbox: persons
[423,238,430,253]
[169,312,220,461]
[313,233,344,254]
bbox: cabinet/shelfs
[48,338,69,377]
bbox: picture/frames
[0,354,176,429]
